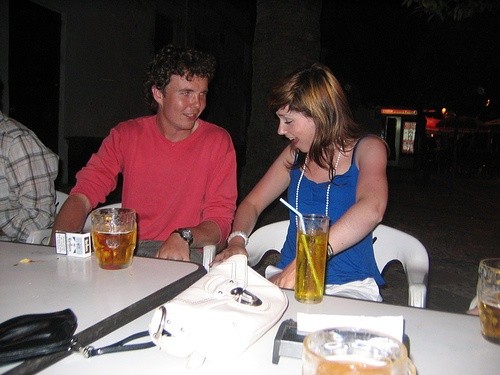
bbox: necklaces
[295,141,346,228]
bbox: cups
[300,326,416,375]
[91,207,138,271]
[476,259,500,344]
[294,213,329,304]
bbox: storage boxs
[55,230,91,258]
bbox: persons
[212,62,390,302]
[49,45,237,265]
[0,79,60,246]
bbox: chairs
[245,220,430,309]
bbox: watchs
[170,228,193,246]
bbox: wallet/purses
[0,308,77,360]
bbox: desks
[0,240,499,375]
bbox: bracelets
[228,231,248,247]
[327,243,333,260]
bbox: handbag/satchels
[150,254,288,366]
